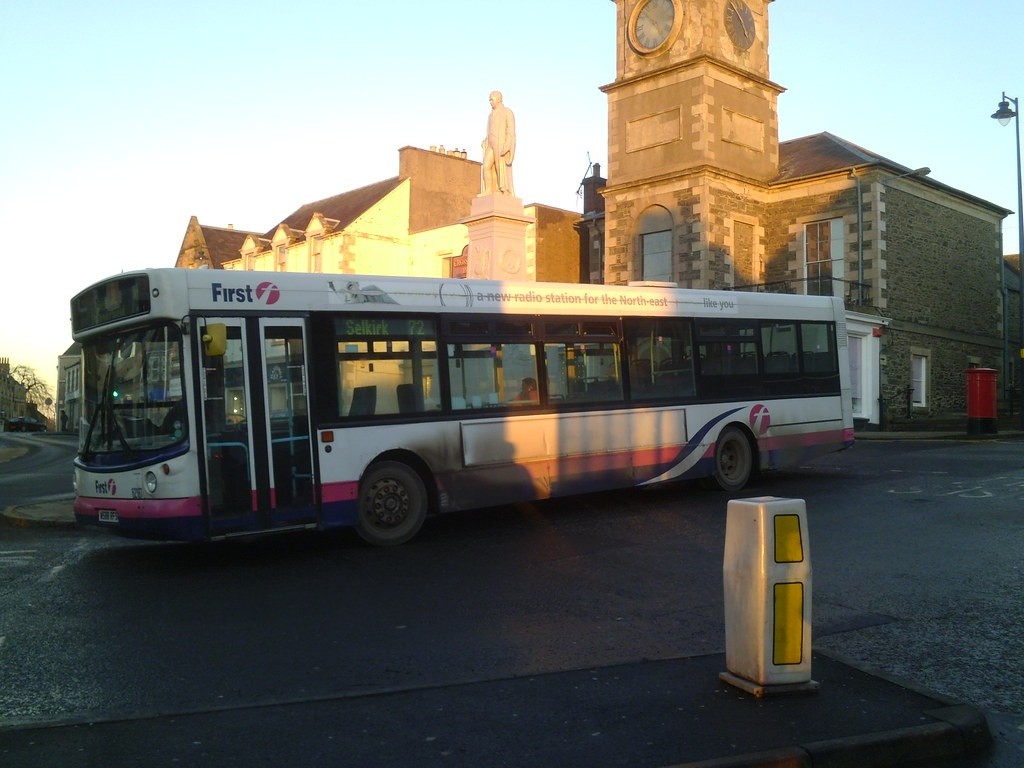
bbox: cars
[8,415,48,433]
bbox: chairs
[608,350,831,385]
[396,384,416,414]
[349,385,378,417]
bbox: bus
[71,268,859,550]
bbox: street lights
[989,90,1024,408]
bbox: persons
[477,91,515,197]
[514,377,537,400]
[60,410,68,431]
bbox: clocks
[634,0,673,48]
[725,0,756,50]
